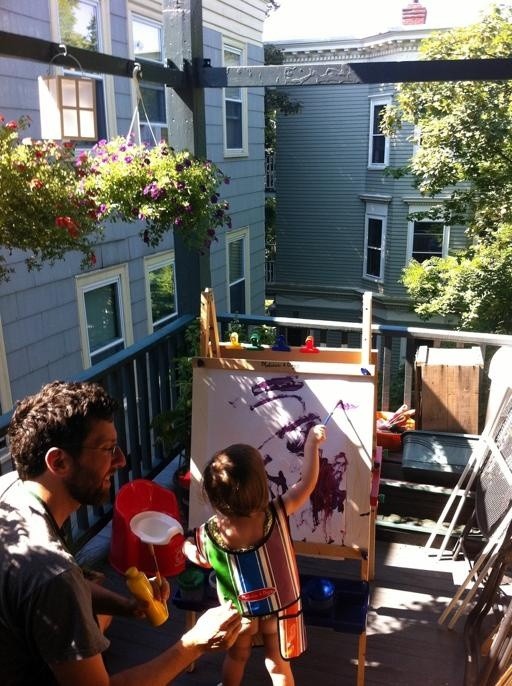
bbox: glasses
[73,444,119,457]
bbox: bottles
[174,570,203,602]
[123,566,170,629]
[303,578,335,608]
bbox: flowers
[1,117,233,287]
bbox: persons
[0,379,244,686]
[179,424,330,686]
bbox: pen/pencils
[148,543,164,593]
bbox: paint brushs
[323,400,343,427]
